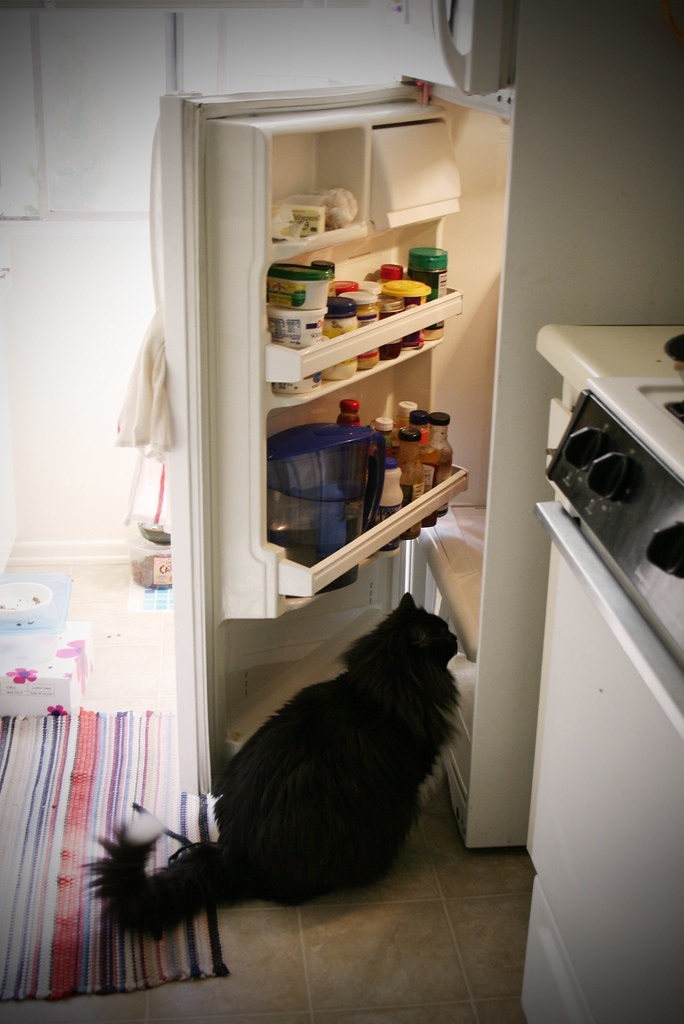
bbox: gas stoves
[536,321,683,661]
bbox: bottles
[390,400,418,463]
[428,411,454,518]
[372,459,404,557]
[397,427,427,537]
[337,399,359,427]
[370,414,395,464]
[407,410,439,526]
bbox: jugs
[267,421,375,598]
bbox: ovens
[513,500,684,1023]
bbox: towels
[109,310,175,461]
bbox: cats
[81,592,465,944]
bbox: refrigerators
[145,0,684,857]
